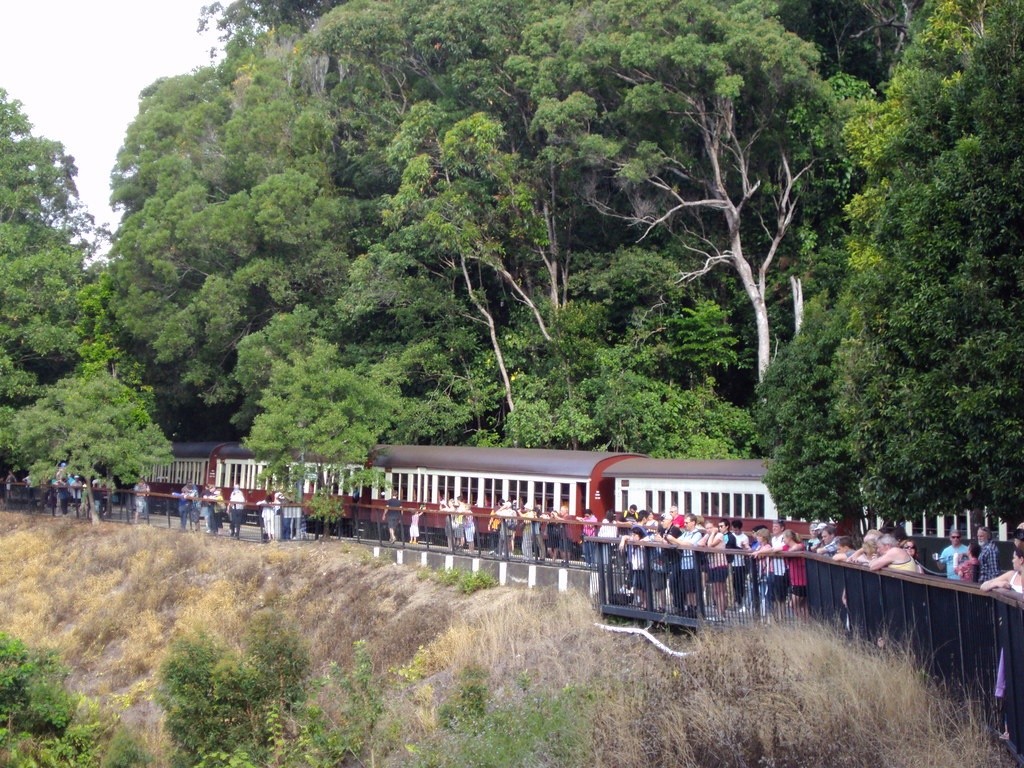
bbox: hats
[632,525,648,536]
[660,513,673,520]
[814,522,827,531]
[810,523,818,531]
[503,502,512,507]
[524,503,532,510]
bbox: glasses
[718,525,725,528]
[903,546,915,549]
[684,522,692,524]
[951,536,961,539]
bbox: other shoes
[389,537,513,558]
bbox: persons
[804,522,856,562]
[932,526,1000,584]
[979,522,1024,739]
[597,505,751,624]
[171,479,245,539]
[382,490,403,543]
[256,495,275,540]
[847,529,925,576]
[133,478,151,525]
[409,505,426,545]
[22,463,108,518]
[489,497,572,567]
[576,509,598,567]
[439,496,476,552]
[740,520,807,625]
[0,469,17,503]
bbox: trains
[149,441,783,530]
